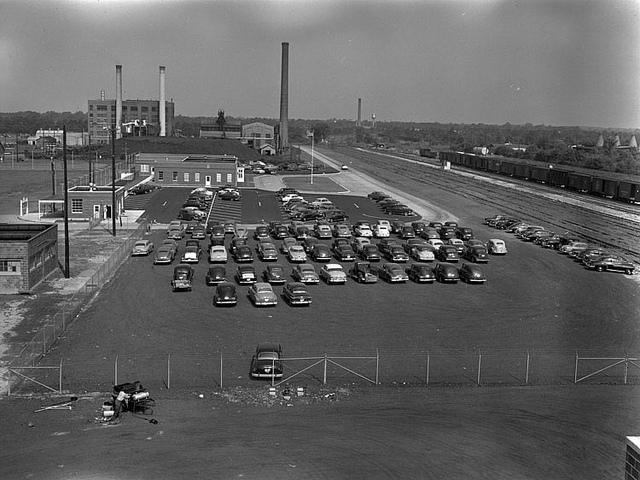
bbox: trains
[437,148,640,207]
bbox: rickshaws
[110,381,159,417]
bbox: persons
[113,389,131,417]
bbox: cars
[153,246,175,265]
[161,182,490,263]
[483,211,636,274]
[239,158,323,176]
[246,282,278,307]
[170,264,194,286]
[145,181,161,190]
[282,280,313,307]
[319,264,348,284]
[248,341,287,380]
[263,264,287,285]
[340,163,349,170]
[211,283,240,308]
[347,262,380,283]
[128,185,150,196]
[290,263,320,284]
[432,263,461,283]
[458,264,487,284]
[205,264,227,285]
[235,263,257,284]
[131,239,154,256]
[377,264,409,283]
[405,263,437,283]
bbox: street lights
[371,110,378,128]
[101,123,123,237]
